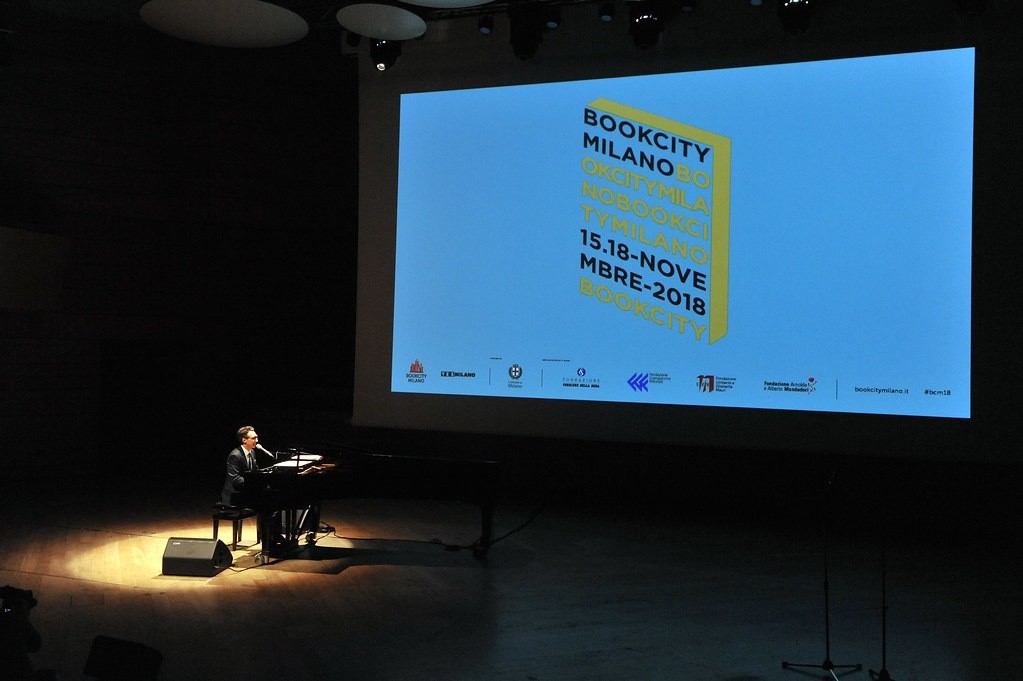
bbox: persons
[0,591,42,681]
[221,426,294,557]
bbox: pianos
[243,453,336,560]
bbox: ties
[247,453,251,471]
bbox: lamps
[599,0,616,23]
[506,0,547,61]
[369,35,402,71]
[626,0,667,51]
[346,30,362,47]
[477,6,495,35]
[546,4,560,28]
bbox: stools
[212,501,261,551]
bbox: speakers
[162,537,232,577]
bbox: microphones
[256,444,275,457]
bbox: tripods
[781,511,895,681]
